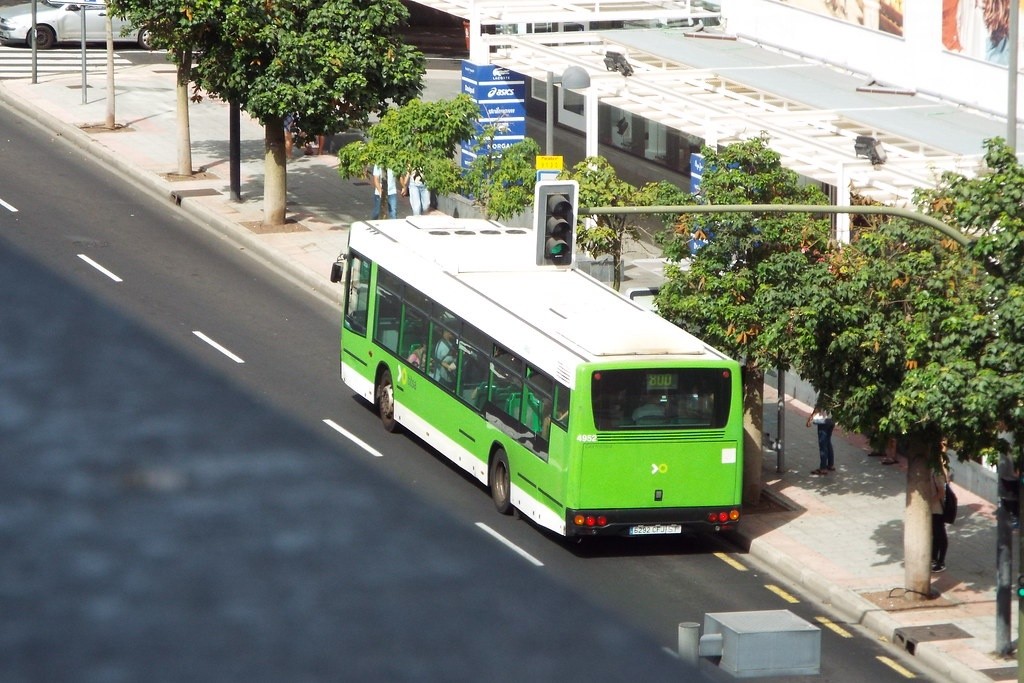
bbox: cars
[0,1,173,51]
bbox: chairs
[409,342,544,434]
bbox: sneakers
[932,559,946,573]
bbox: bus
[329,210,748,543]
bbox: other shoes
[812,464,835,474]
[868,451,887,456]
[881,456,899,464]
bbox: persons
[928,460,950,572]
[370,158,406,220]
[997,442,1018,528]
[347,273,716,456]
[402,158,432,216]
[807,391,838,476]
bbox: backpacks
[943,483,957,524]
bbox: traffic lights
[534,180,579,271]
